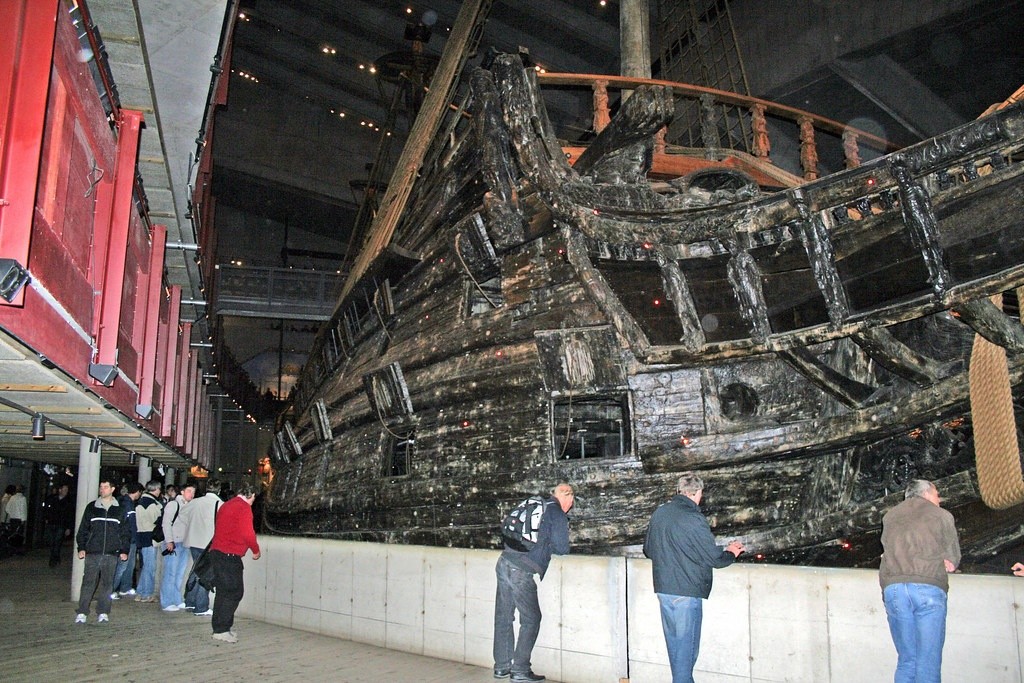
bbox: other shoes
[135,593,160,604]
[162,603,188,612]
[120,588,137,595]
[194,608,214,616]
[213,630,239,643]
[111,592,120,601]
[50,558,61,567]
[186,606,195,611]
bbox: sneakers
[98,613,110,623]
[76,613,87,624]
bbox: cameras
[162,549,172,555]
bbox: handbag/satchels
[195,551,218,592]
[7,525,25,547]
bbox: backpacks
[151,499,179,542]
[499,495,556,552]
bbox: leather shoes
[510,670,545,682]
[495,669,511,678]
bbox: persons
[880,480,961,683]
[76,479,131,622]
[111,478,225,616]
[0,484,27,556]
[493,484,574,683]
[46,484,73,567]
[211,481,262,643]
[643,475,745,683]
